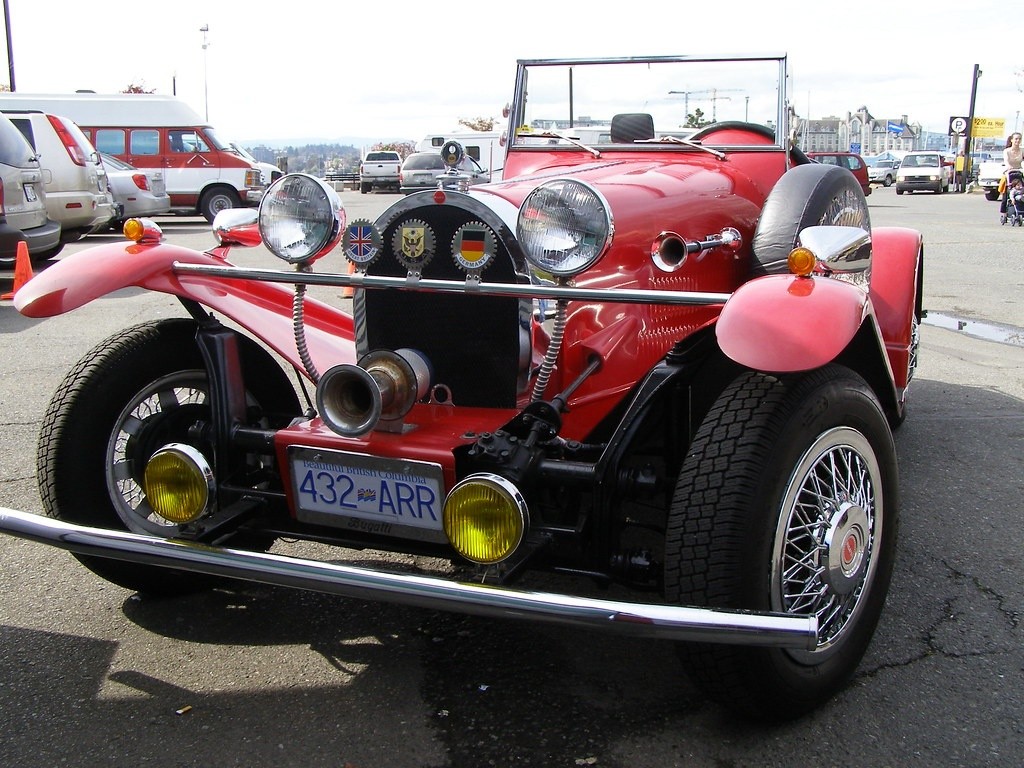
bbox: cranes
[668,88,742,126]
[684,97,733,122]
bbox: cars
[0,48,930,731]
[398,151,491,197]
[0,111,62,266]
[0,106,124,234]
[804,151,873,199]
[99,152,171,230]
[866,158,900,186]
[894,150,953,196]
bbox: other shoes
[955,190,959,192]
[1000,216,1008,223]
[964,190,966,192]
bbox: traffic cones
[0,240,41,302]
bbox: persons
[1009,179,1024,205]
[955,150,966,192]
[940,156,955,168]
[1003,133,1024,171]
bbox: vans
[179,139,286,193]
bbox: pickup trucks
[359,150,402,195]
[979,154,1006,202]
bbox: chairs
[908,157,918,165]
[610,111,655,143]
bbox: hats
[1012,179,1020,187]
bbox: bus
[0,92,263,225]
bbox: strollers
[1001,170,1024,227]
